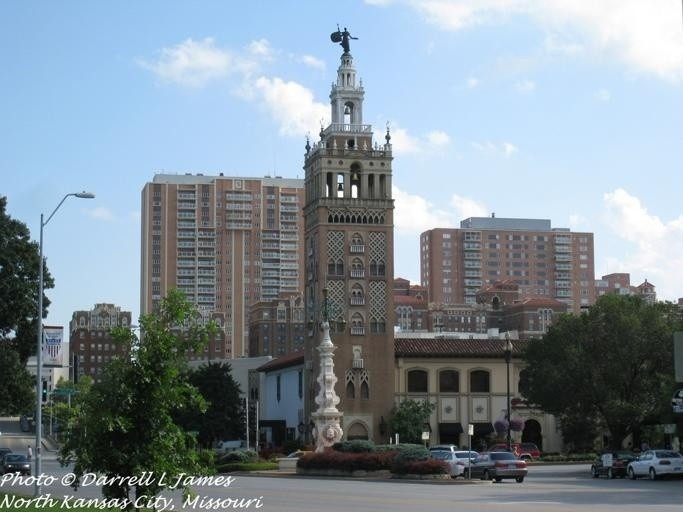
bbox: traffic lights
[42,381,47,401]
[234,398,247,441]
[248,399,257,432]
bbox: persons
[628,442,635,451]
[27,446,32,462]
[641,439,651,450]
[513,446,518,455]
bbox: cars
[590,449,683,481]
[288,448,314,459]
[429,443,540,482]
[0,447,31,476]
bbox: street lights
[34,191,95,498]
[322,288,329,322]
[502,329,513,451]
